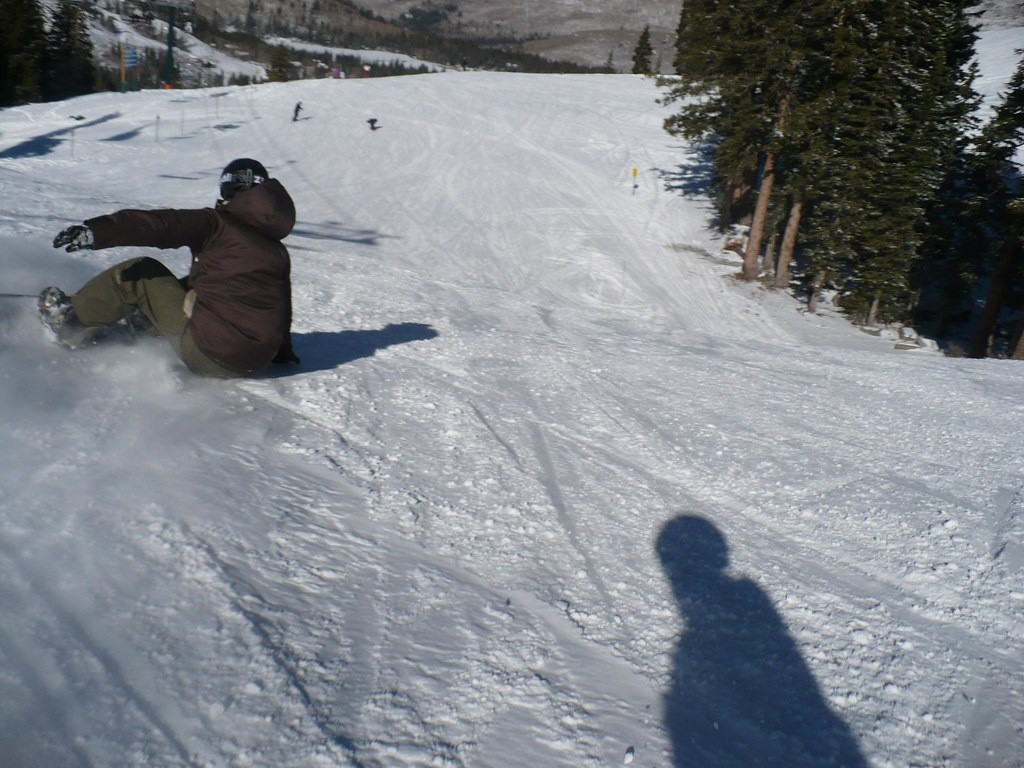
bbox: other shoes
[38,287,86,350]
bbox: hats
[221,158,268,201]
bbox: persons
[330,64,371,78]
[293,101,304,121]
[368,118,378,129]
[37,158,298,379]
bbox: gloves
[272,332,300,366]
[53,225,94,252]
